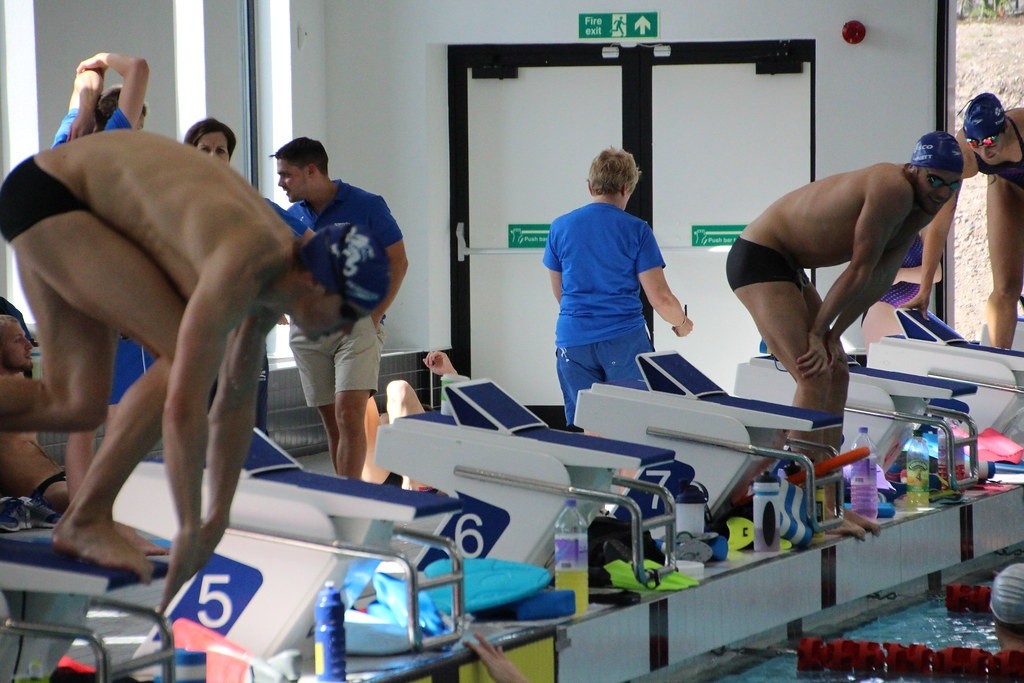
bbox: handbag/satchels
[588,515,665,587]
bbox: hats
[301,221,387,309]
[990,563,1024,625]
[911,131,965,170]
[962,92,1006,138]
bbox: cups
[440,373,470,417]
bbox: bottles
[314,581,347,683]
[907,430,930,511]
[851,426,878,522]
[553,497,589,618]
[752,470,782,552]
[963,454,995,481]
[937,417,966,491]
[675,486,706,539]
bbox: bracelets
[679,316,686,329]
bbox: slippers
[660,527,718,566]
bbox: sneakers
[1,490,65,532]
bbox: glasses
[966,134,1000,147]
[338,302,361,321]
[923,168,962,190]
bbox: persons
[990,563,1024,653]
[269,136,408,479]
[725,132,964,541]
[542,145,694,426]
[898,93,1024,347]
[860,231,942,350]
[50,52,154,434]
[361,351,459,494]
[0,297,68,514]
[0,130,390,614]
[184,119,317,437]
